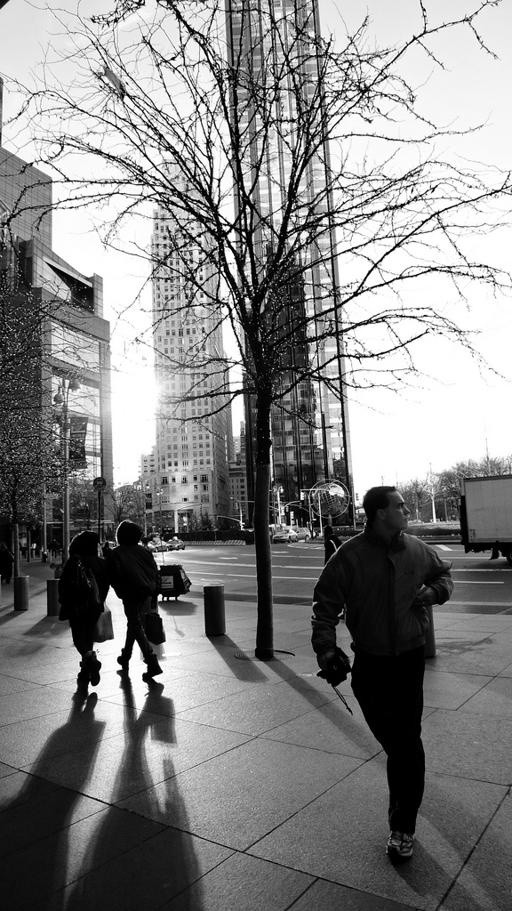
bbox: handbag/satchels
[95,611,114,642]
[141,612,166,645]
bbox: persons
[57,530,121,686]
[113,519,166,678]
[323,525,342,565]
[309,485,454,858]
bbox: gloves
[315,648,352,687]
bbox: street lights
[231,496,243,529]
[52,377,71,569]
[273,485,284,523]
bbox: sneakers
[385,830,414,857]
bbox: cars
[147,536,185,552]
[272,527,316,544]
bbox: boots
[77,650,102,686]
[142,654,163,678]
[142,679,163,699]
[117,669,132,688]
[117,648,132,665]
[72,687,98,711]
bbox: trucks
[458,473,512,561]
